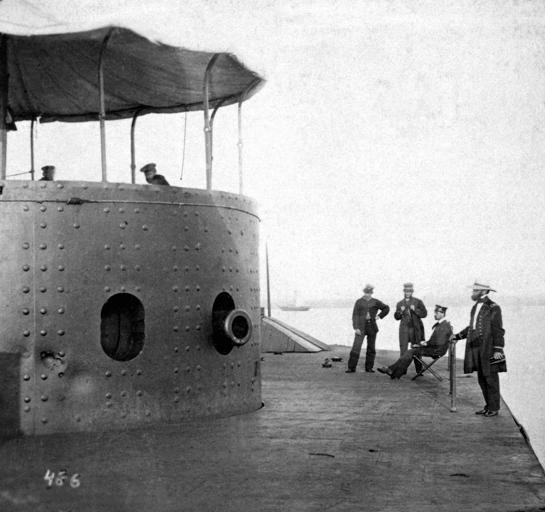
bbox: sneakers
[345,366,423,379]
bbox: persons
[377,304,452,379]
[393,282,428,376]
[448,282,507,417]
[40,165,57,182]
[139,162,169,186]
[344,285,391,373]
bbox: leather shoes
[475,409,499,418]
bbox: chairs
[410,326,454,382]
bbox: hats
[139,163,157,173]
[363,284,374,294]
[41,166,55,171]
[465,278,498,293]
[402,281,414,291]
[433,303,448,314]
